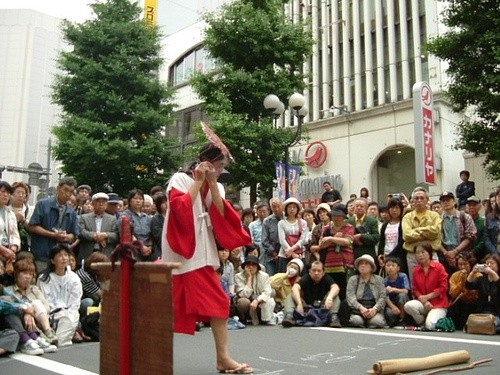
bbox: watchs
[21,304,28,311]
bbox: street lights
[262,91,309,202]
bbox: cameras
[477,264,485,272]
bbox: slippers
[220,363,254,374]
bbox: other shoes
[77,328,92,341]
[267,312,277,325]
[330,313,341,327]
[282,313,296,327]
[277,311,284,324]
[73,331,83,342]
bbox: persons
[455,170,475,209]
[162,145,254,374]
[217,197,340,326]
[350,187,371,202]
[321,182,342,208]
[0,176,119,356]
[320,195,451,331]
[119,185,167,261]
[402,186,441,298]
[430,186,500,334]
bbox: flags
[201,121,229,155]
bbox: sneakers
[21,339,44,355]
[36,337,57,352]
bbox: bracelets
[373,306,379,309]
[454,247,459,253]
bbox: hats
[467,195,481,203]
[282,197,303,213]
[439,192,454,201]
[287,258,304,274]
[107,193,119,203]
[315,203,331,220]
[482,196,489,206]
[354,254,376,273]
[326,203,348,220]
[241,256,262,270]
[91,192,109,202]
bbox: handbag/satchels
[466,313,497,334]
[293,305,329,327]
